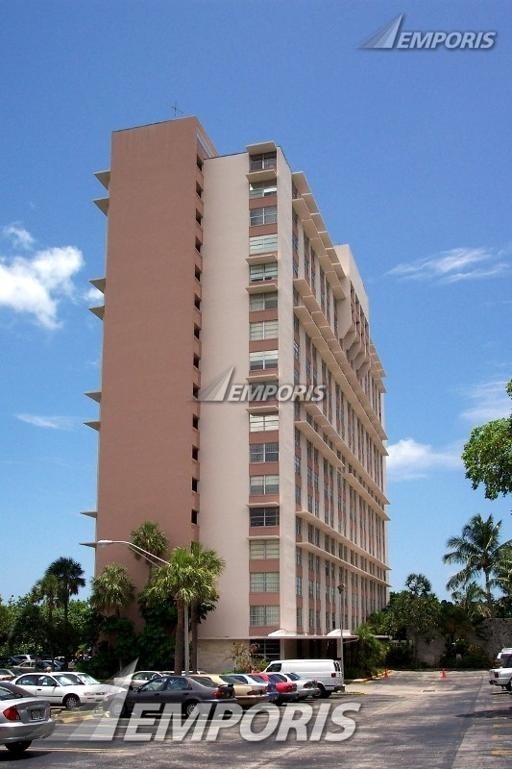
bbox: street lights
[94,539,192,674]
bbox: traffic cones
[441,670,447,679]
[382,668,389,679]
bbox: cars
[114,658,344,717]
[489,647,512,690]
[0,652,125,755]
